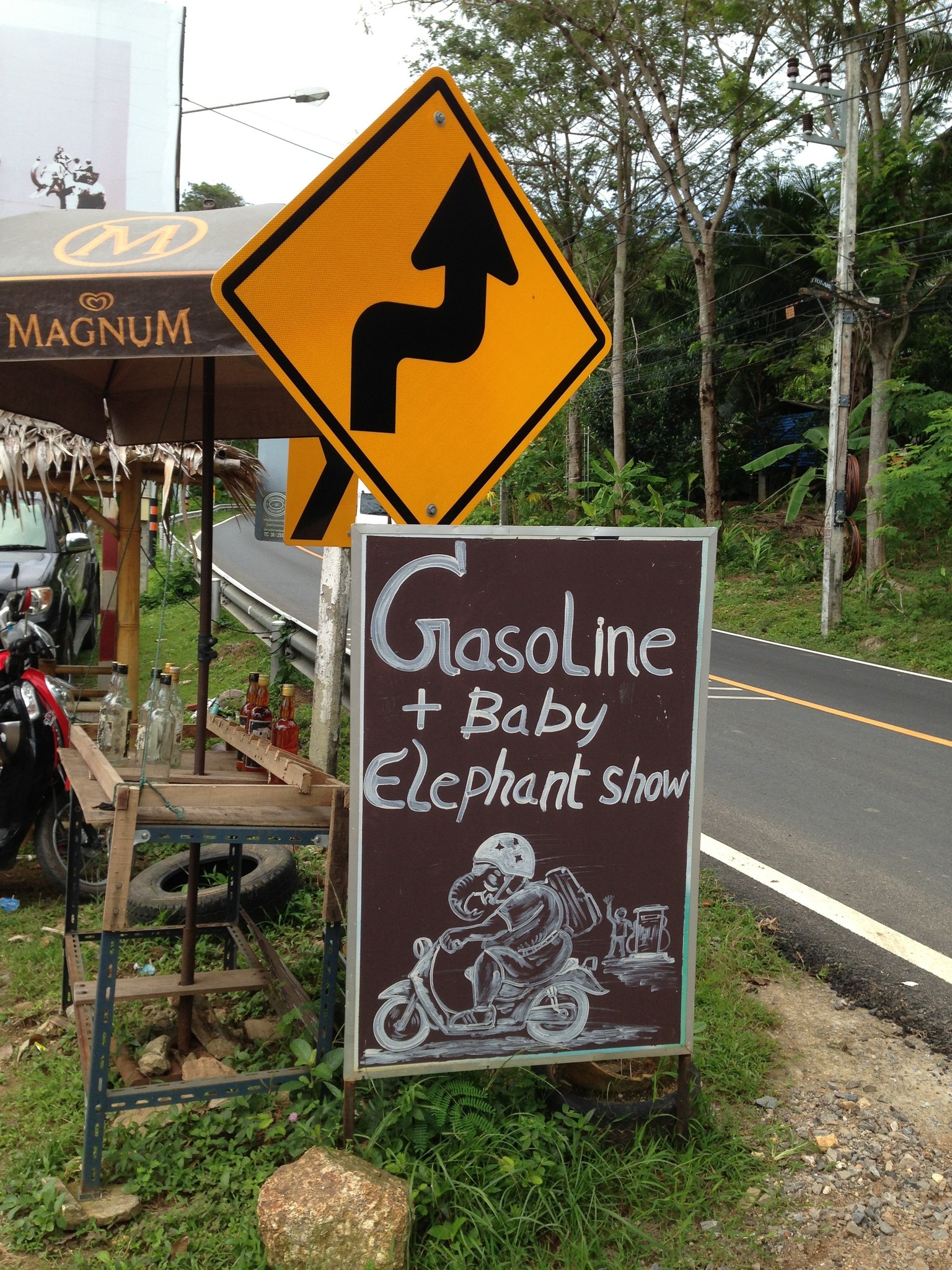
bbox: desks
[55,714,351,1203]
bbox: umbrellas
[1,200,321,1053]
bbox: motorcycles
[0,565,111,902]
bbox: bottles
[237,671,259,772]
[164,662,175,674]
[134,667,162,768]
[268,683,299,785]
[242,674,273,772]
[169,666,184,769]
[103,663,133,768]
[96,661,123,754]
[144,673,176,784]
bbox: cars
[0,490,101,666]
